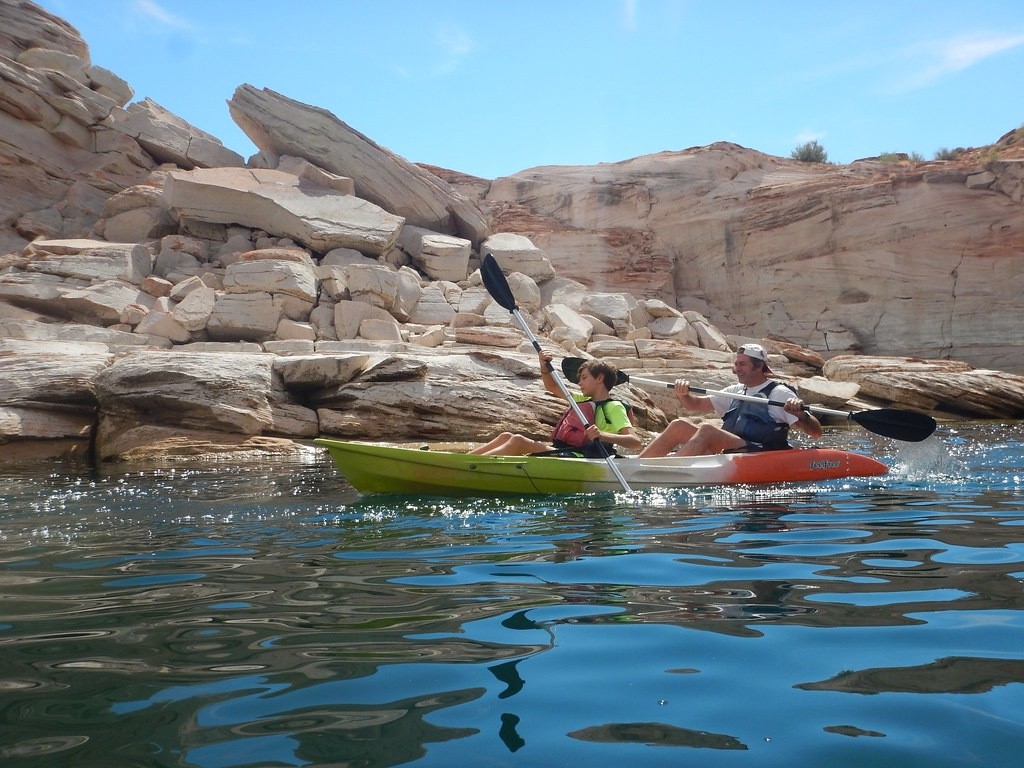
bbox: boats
[311,435,892,493]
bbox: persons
[636,344,822,458]
[467,349,639,455]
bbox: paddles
[558,354,940,445]
[478,250,637,496]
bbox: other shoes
[615,454,629,459]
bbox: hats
[736,343,774,374]
